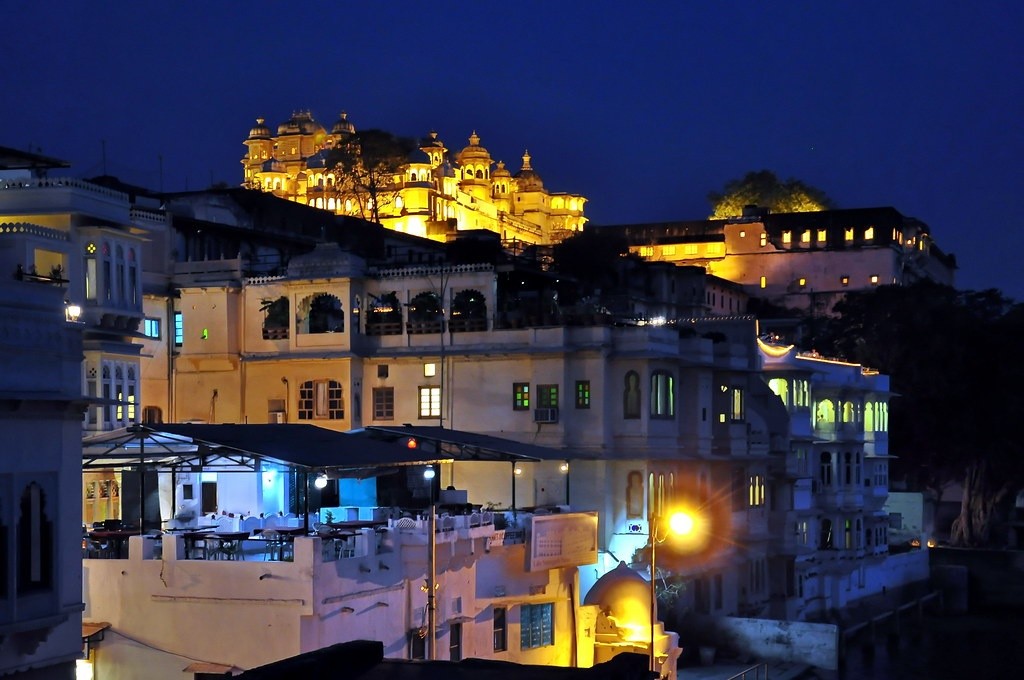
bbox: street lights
[648,508,695,671]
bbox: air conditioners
[533,408,558,423]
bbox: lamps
[68,306,81,321]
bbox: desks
[276,529,315,561]
[89,531,141,558]
[331,520,387,528]
[184,532,249,559]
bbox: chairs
[185,531,250,561]
[262,528,386,560]
[82,519,135,560]
